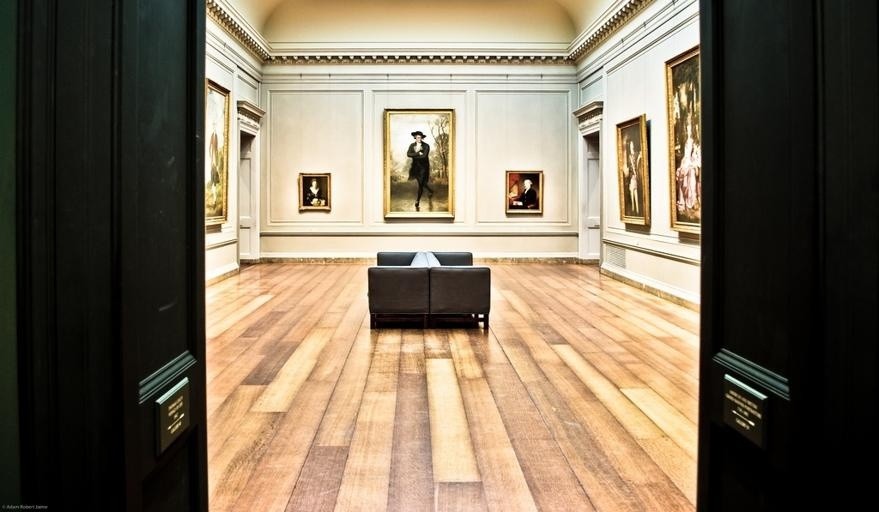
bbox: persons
[625,140,641,215]
[406,131,435,208]
[675,91,702,215]
[512,178,538,209]
[305,179,326,207]
[208,122,220,210]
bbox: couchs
[366,249,493,332]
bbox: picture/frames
[204,76,232,227]
[380,106,456,222]
[503,169,543,217]
[296,171,333,213]
[615,44,700,236]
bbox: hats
[411,131,426,139]
